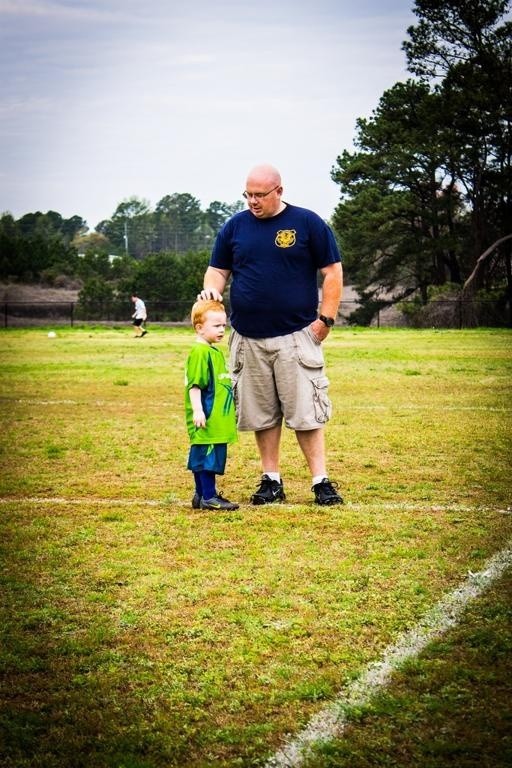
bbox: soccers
[47,332,55,337]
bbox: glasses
[242,186,277,202]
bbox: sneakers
[192,493,239,511]
[314,478,343,505]
[135,331,147,337]
[251,474,286,505]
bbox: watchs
[319,314,334,326]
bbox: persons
[197,165,344,504]
[130,293,147,337]
[184,298,239,509]
